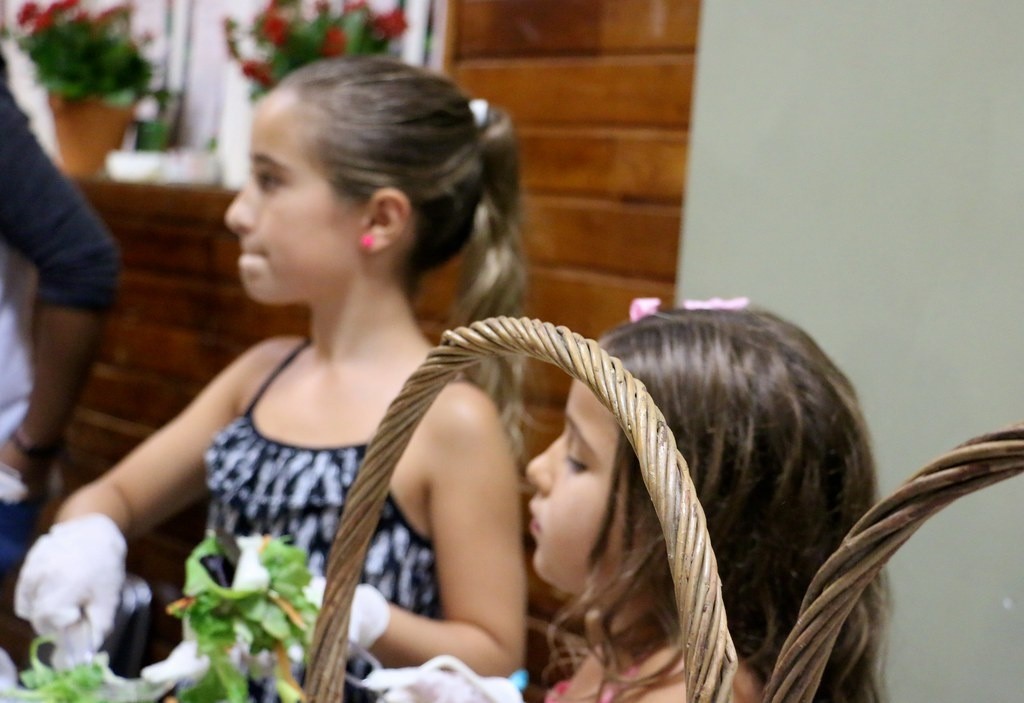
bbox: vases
[48,89,131,175]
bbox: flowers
[218,0,411,104]
[16,0,170,107]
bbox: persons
[13,58,531,703]
[525,307,896,702]
[0,62,121,621]
